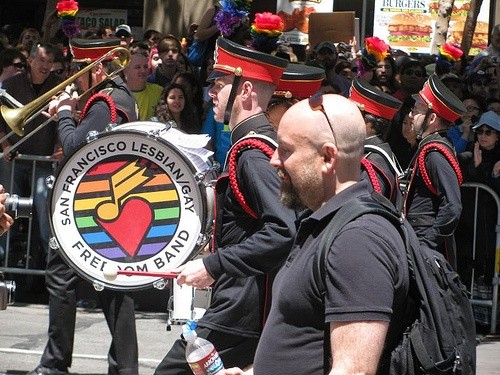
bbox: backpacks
[315,193,477,375]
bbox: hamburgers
[387,0,490,56]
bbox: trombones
[0,47,132,160]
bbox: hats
[439,73,460,82]
[479,111,500,131]
[56,0,121,75]
[250,11,325,108]
[116,24,131,34]
[316,41,336,53]
[471,70,492,81]
[411,42,467,122]
[348,36,402,119]
[206,0,288,81]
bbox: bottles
[182,320,226,375]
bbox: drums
[47,121,218,292]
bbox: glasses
[116,31,130,38]
[50,69,64,75]
[472,81,489,87]
[310,92,337,149]
[411,108,430,115]
[477,129,496,136]
[9,63,26,69]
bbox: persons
[405,43,467,271]
[0,0,500,334]
[348,38,406,211]
[231,94,411,375]
[153,1,298,375]
[27,0,138,375]
[252,13,326,130]
